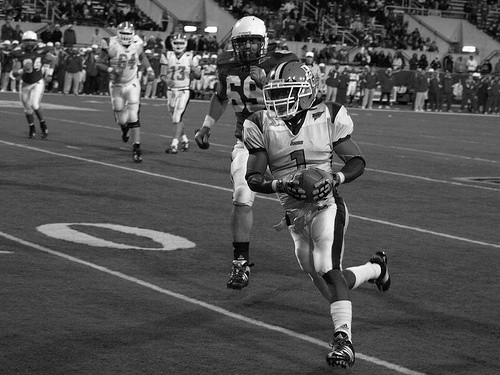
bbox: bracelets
[202,115,216,129]
[337,172,344,184]
[272,179,281,193]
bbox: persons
[11,31,57,138]
[243,58,391,369]
[138,31,233,99]
[94,21,155,162]
[159,32,202,154]
[214,0,500,51]
[194,16,299,289]
[0,16,109,96]
[301,44,406,109]
[0,0,158,30]
[409,53,500,115]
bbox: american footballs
[287,169,329,203]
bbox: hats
[1,40,98,54]
[305,52,314,58]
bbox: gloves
[194,126,211,149]
[250,66,266,89]
[311,168,340,202]
[276,168,306,203]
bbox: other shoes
[41,124,48,138]
[133,152,142,162]
[29,128,35,138]
[122,125,130,142]
[180,141,189,152]
[165,146,179,154]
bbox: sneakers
[327,331,355,368]
[370,251,391,291]
[227,260,254,289]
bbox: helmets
[22,30,38,41]
[231,16,269,64]
[116,21,135,45]
[171,35,188,51]
[264,60,317,111]
